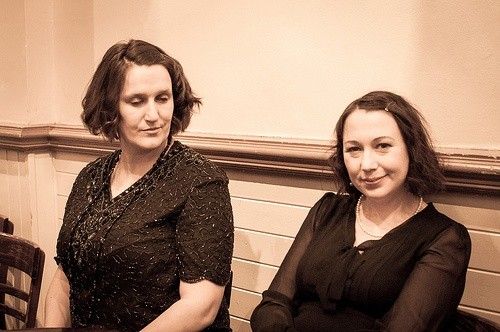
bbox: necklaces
[356,192,423,237]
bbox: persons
[249,91,472,332]
[43,40,235,332]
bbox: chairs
[0,231,45,332]
[449,303,500,331]
[0,214,15,329]
